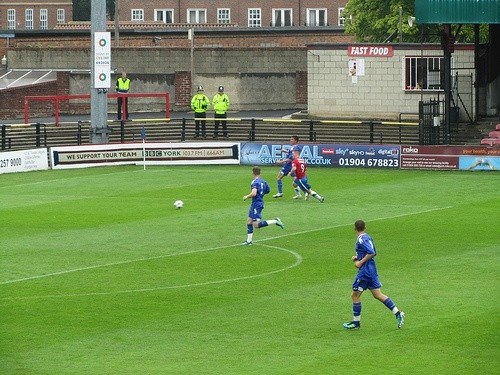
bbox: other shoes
[223,135,229,137]
[193,135,199,138]
[213,133,218,138]
[202,135,206,138]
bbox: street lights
[1,55,8,70]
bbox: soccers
[174,200,184,208]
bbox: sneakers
[320,196,324,203]
[273,193,283,197]
[293,195,302,199]
[343,321,360,329]
[242,240,253,246]
[396,312,405,328]
[275,217,284,229]
[304,192,309,200]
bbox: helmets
[219,86,224,92]
[198,85,204,90]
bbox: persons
[211,86,230,140]
[343,220,405,330]
[241,167,285,246]
[190,86,211,139]
[272,136,302,198]
[116,72,131,120]
[288,149,324,204]
